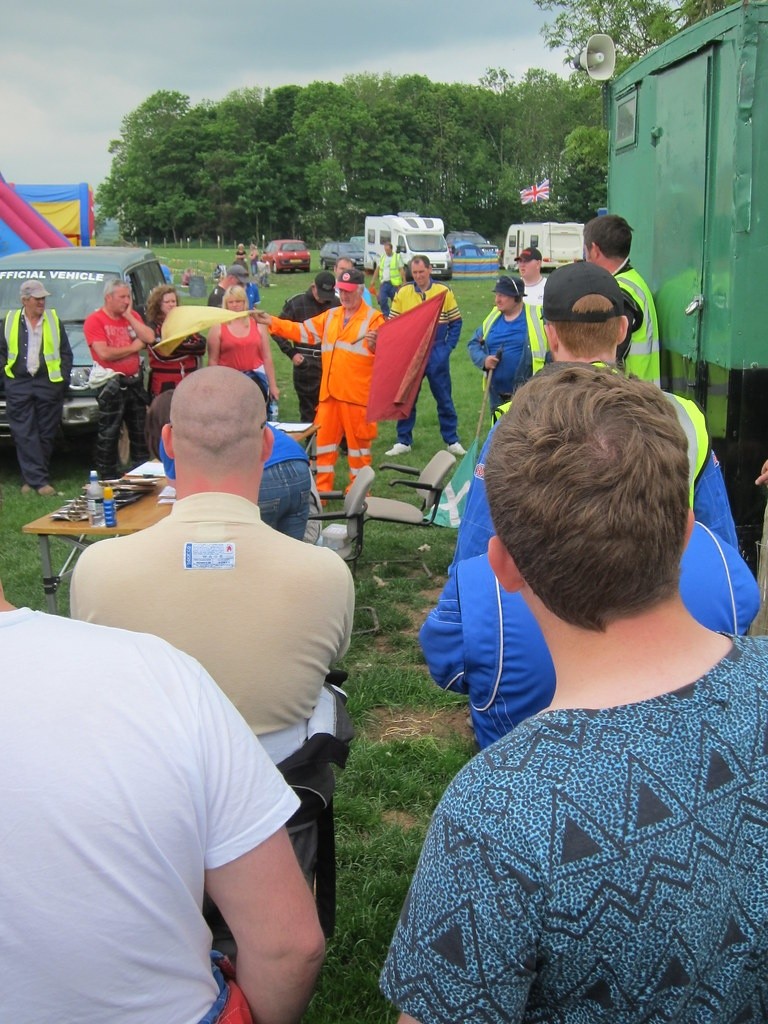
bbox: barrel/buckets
[188,276,206,298]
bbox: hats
[493,276,527,297]
[227,264,250,283]
[334,270,364,293]
[514,247,541,262]
[20,280,50,299]
[540,260,624,322]
[315,272,336,302]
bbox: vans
[0,246,166,476]
[349,236,365,248]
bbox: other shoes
[21,484,31,494]
[38,485,55,496]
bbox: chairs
[308,464,380,636]
[353,449,457,581]
[201,669,354,939]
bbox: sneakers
[447,442,467,456]
[385,442,411,456]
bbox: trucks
[445,229,499,272]
[503,222,585,271]
[364,211,455,283]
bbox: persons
[0,214,768,752]
[69,365,355,817]
[0,280,74,496]
[83,277,156,480]
[379,361,768,1024]
[248,270,388,506]
[0,564,324,1024]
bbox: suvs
[259,239,310,275]
[319,242,365,271]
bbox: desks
[23,426,320,615]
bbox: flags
[519,177,551,206]
[422,436,480,527]
[366,290,448,421]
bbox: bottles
[269,398,278,422]
[103,487,117,527]
[86,470,105,528]
